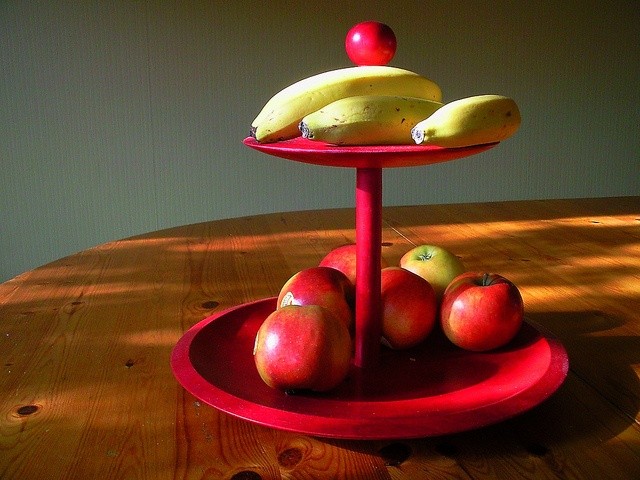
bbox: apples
[380,266,438,353]
[397,244,469,305]
[252,303,355,396]
[318,244,356,287]
[438,270,525,354]
[277,266,356,341]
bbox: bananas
[410,94,523,148]
[250,65,442,144]
[297,94,446,144]
